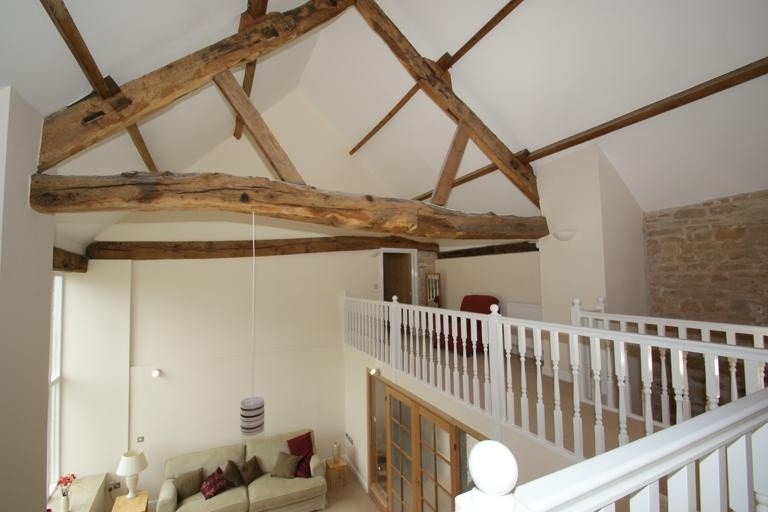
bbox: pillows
[270,430,314,478]
[173,453,264,500]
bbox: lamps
[116,452,148,498]
[239,212,265,437]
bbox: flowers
[57,473,77,495]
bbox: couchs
[154,428,328,512]
[432,296,501,355]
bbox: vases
[60,496,69,511]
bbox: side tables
[111,490,149,512]
[325,458,348,489]
